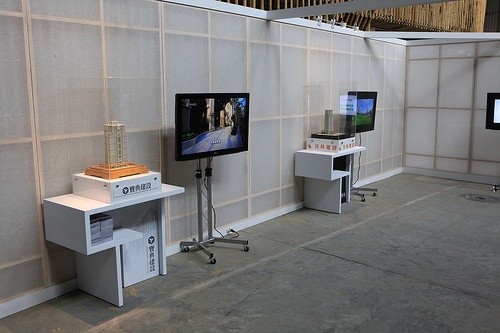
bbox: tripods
[180,156,249,263]
[348,134,379,203]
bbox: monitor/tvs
[174,92,250,161]
[485,92,500,130]
[345,92,378,134]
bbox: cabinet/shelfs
[43,170,186,307]
[294,133,367,214]
